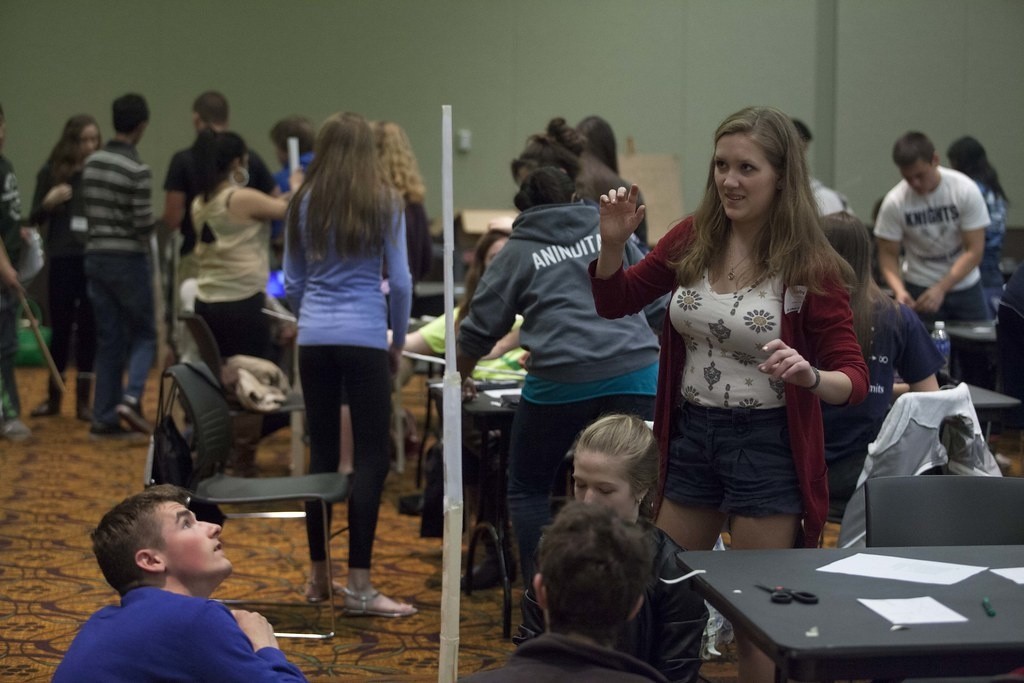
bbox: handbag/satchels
[142,415,196,494]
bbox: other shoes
[90,422,117,435]
[460,552,519,590]
[116,401,148,434]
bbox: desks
[425,379,521,637]
[676,546,1024,682]
[960,379,1021,450]
[947,319,1005,391]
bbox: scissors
[755,584,819,604]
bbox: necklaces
[728,239,754,280]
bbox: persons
[388,107,1024,683]
[162,92,431,618]
[50,483,309,683]
[30,93,168,437]
[0,103,33,438]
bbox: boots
[31,376,65,418]
[75,377,97,422]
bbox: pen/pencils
[983,598,995,618]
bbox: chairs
[177,311,305,472]
[865,475,1024,546]
[838,380,1004,547]
[163,362,350,640]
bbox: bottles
[930,321,950,379]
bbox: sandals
[342,589,418,616]
[309,583,353,603]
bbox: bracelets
[805,366,820,390]
